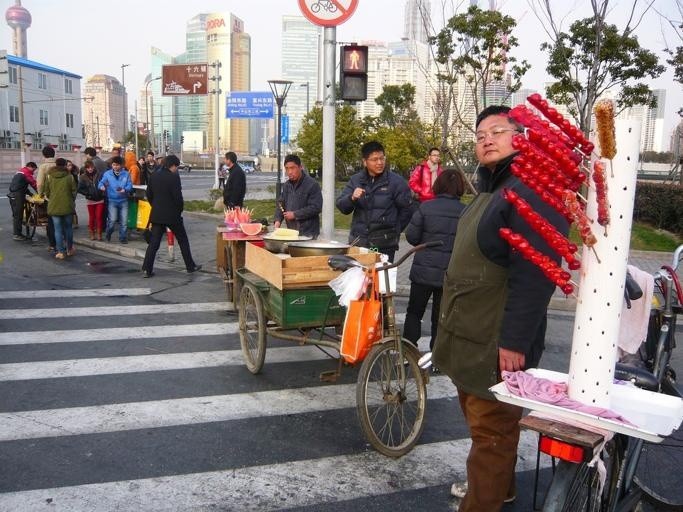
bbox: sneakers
[452,482,516,503]
[12,229,202,278]
[394,357,409,366]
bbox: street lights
[144,75,163,163]
[120,63,130,151]
[268,80,292,208]
[300,81,310,124]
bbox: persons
[217,163,226,189]
[35,145,202,279]
[273,156,321,238]
[430,105,575,512]
[336,141,409,262]
[222,151,245,209]
[393,170,468,373]
[407,148,444,204]
[5,162,37,241]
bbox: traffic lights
[341,44,367,101]
[163,130,166,140]
[179,135,184,144]
[166,130,168,139]
[165,145,169,153]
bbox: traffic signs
[278,116,289,143]
[225,92,272,118]
[162,64,206,95]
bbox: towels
[618,262,656,353]
[500,367,642,429]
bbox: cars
[221,162,255,175]
[178,161,193,174]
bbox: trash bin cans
[127,185,152,239]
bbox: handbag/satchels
[369,230,398,251]
[409,164,423,200]
[341,301,384,365]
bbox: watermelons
[240,223,263,235]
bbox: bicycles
[516,241,682,510]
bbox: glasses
[472,126,519,143]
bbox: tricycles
[21,193,74,246]
[233,236,443,456]
